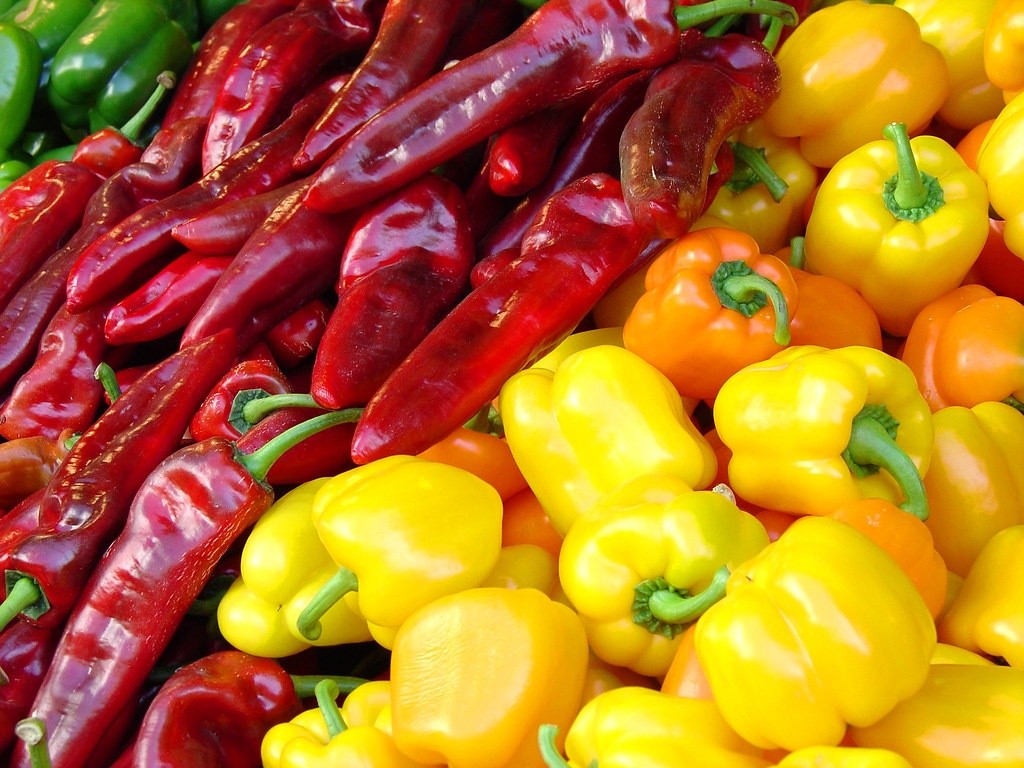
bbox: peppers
[0,0,1024,768]
